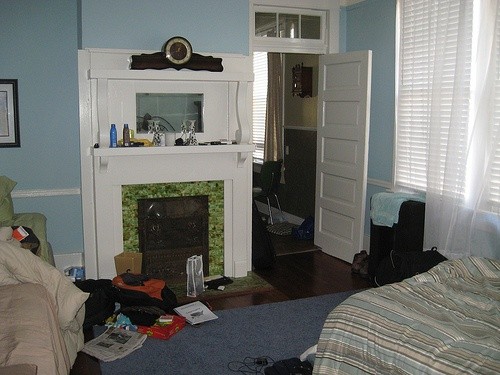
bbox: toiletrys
[122,123,132,147]
[109,123,119,148]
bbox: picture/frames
[0,78,21,148]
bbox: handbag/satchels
[384,247,440,279]
[185,254,208,297]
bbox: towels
[368,189,421,229]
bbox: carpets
[89,290,376,375]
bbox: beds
[313,255,499,374]
[0,256,58,374]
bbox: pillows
[0,175,18,222]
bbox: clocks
[165,36,192,65]
